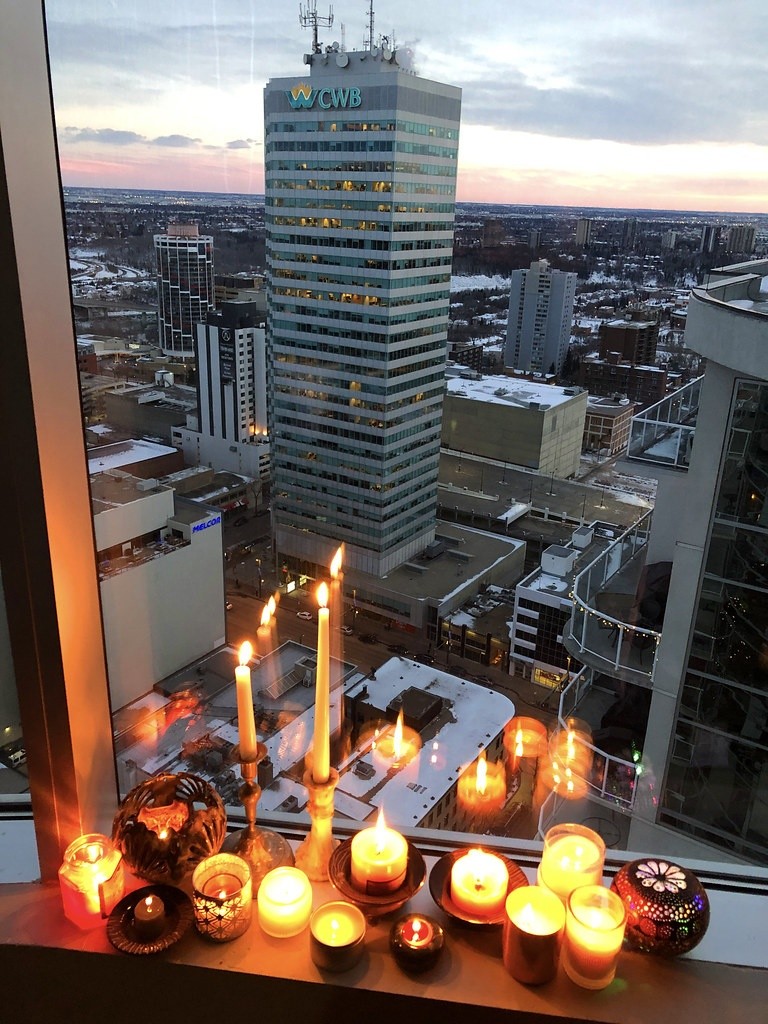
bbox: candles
[428,823,630,987]
[372,727,416,779]
[256,626,278,702]
[455,716,597,816]
[60,580,440,973]
[330,578,340,747]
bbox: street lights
[256,559,261,598]
[352,589,356,629]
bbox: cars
[334,625,353,636]
[226,602,232,610]
[414,654,436,666]
[388,645,408,655]
[297,612,312,621]
